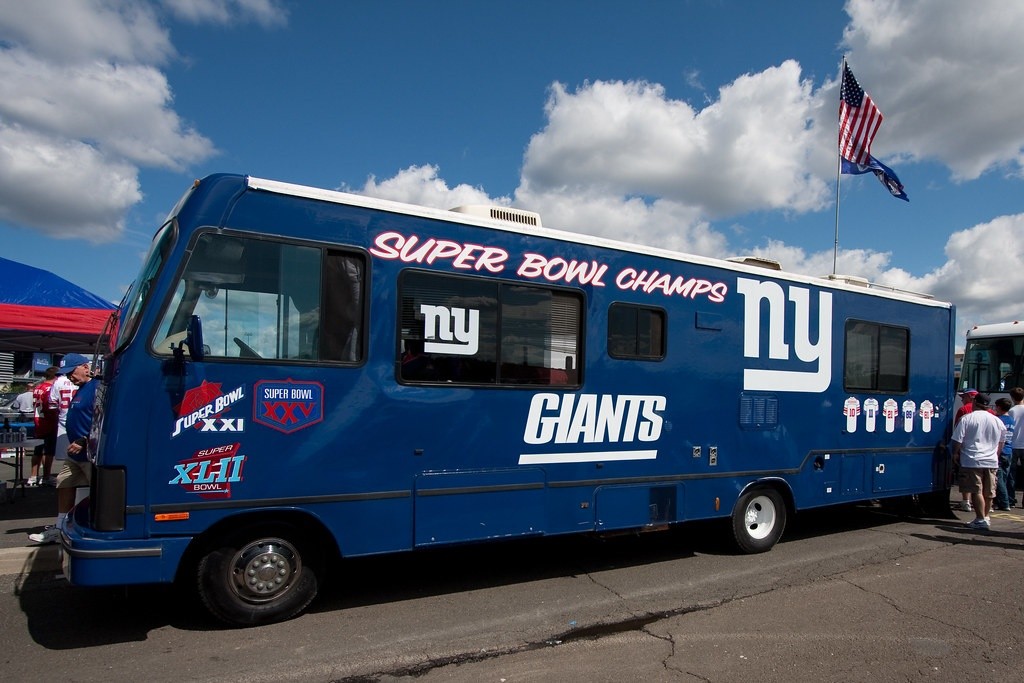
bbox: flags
[837,58,910,203]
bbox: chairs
[290,259,361,362]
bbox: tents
[0,257,124,353]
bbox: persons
[16,383,34,423]
[27,366,63,486]
[49,375,79,474]
[28,352,99,544]
[950,386,1024,529]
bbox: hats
[958,387,978,400]
[54,354,89,377]
[975,392,992,408]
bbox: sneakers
[28,521,63,545]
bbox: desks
[0,439,44,505]
[0,422,35,437]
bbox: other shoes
[26,475,39,486]
[990,508,994,512]
[1001,507,1011,511]
[39,476,58,486]
[1012,504,1016,508]
[954,502,963,508]
[960,504,973,512]
[964,518,990,530]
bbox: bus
[953,320,1024,469]
[55,171,957,629]
